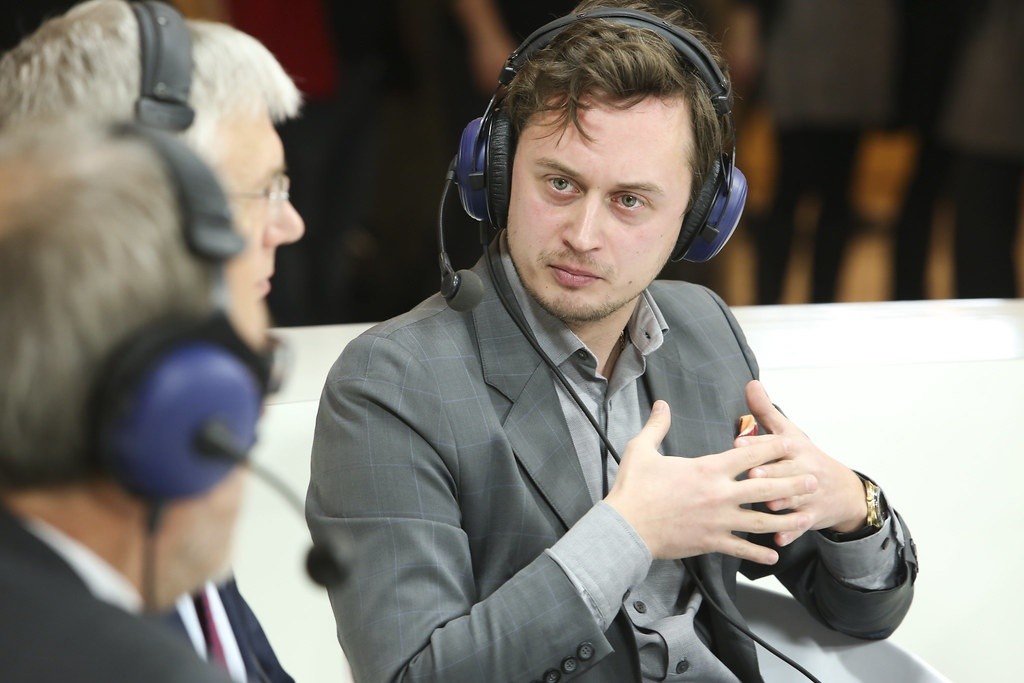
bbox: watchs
[822,468,888,540]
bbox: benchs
[222,297,1024,683]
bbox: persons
[305,0,918,683]
[0,0,302,683]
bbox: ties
[189,588,235,681]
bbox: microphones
[438,153,484,312]
[229,451,359,587]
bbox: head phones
[85,116,270,504]
[456,7,747,263]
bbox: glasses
[228,173,291,209]
